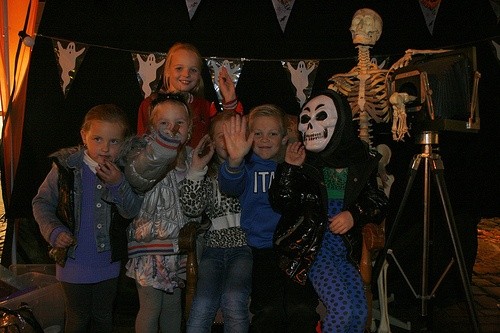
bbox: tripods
[371,132,481,333]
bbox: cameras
[388,46,480,132]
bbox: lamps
[18,31,35,47]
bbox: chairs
[178,115,386,333]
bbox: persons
[136,41,244,152]
[268,88,390,333]
[122,94,203,333]
[217,103,322,333]
[325,6,419,199]
[179,108,254,333]
[32,102,145,333]
